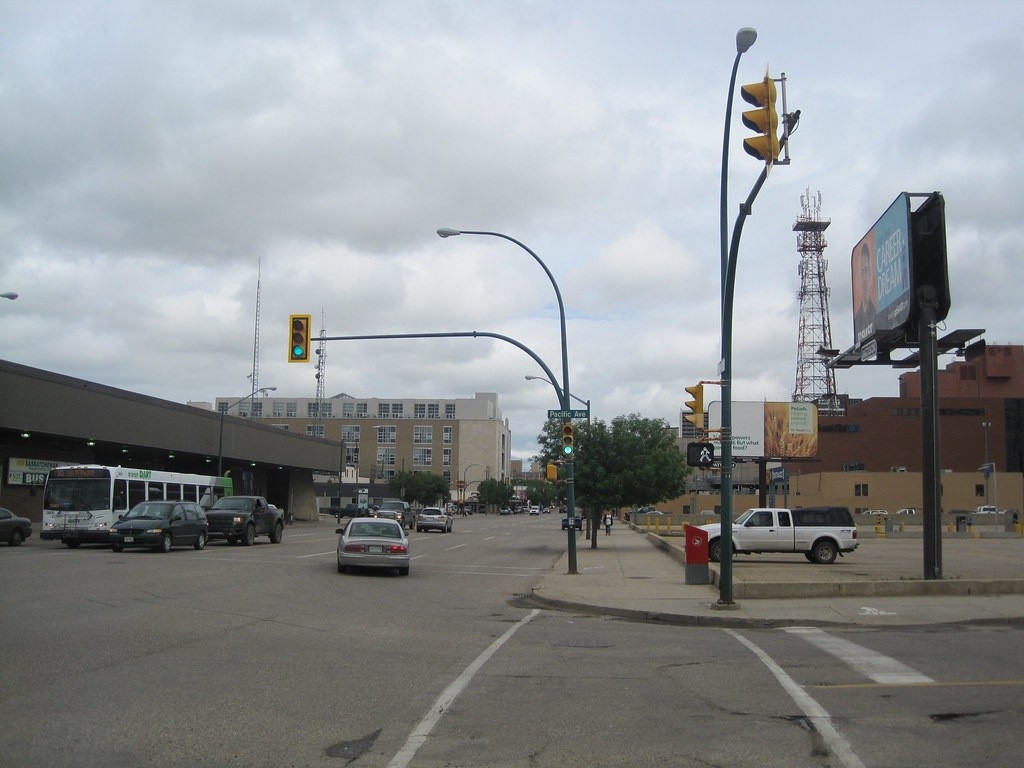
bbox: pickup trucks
[696,506,859,564]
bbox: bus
[40,464,233,549]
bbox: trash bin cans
[884,518,893,533]
[684,523,709,585]
[956,515,966,531]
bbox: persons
[967,513,973,526]
[353,524,362,534]
[464,507,467,516]
[1013,509,1020,524]
[603,512,613,535]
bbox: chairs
[353,527,365,534]
[381,529,394,535]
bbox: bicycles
[284,510,294,525]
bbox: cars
[417,504,473,533]
[109,501,209,553]
[0,507,33,547]
[500,505,568,515]
[205,496,284,546]
[335,518,410,576]
[561,515,584,531]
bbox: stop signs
[457,481,465,488]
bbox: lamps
[249,461,256,466]
[203,455,212,462]
[86,440,96,446]
[314,469,320,473]
[120,444,129,453]
[167,449,175,458]
[19,430,31,438]
[276,464,284,470]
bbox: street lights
[982,421,991,506]
[525,375,591,438]
[437,228,580,575]
[711,26,758,612]
[458,461,484,518]
[218,387,277,476]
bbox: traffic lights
[685,385,704,431]
[563,423,573,456]
[741,69,779,165]
[288,314,311,363]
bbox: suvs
[329,501,416,530]
[624,506,665,521]
